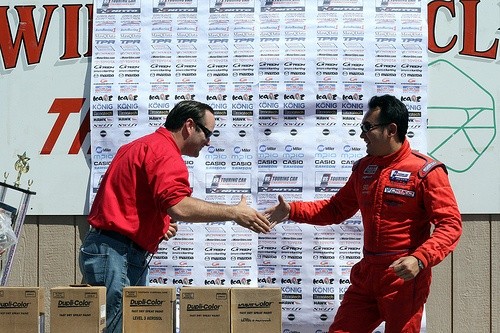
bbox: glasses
[360,122,393,132]
[199,125,214,141]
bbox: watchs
[418,259,424,270]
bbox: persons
[261,94,462,333]
[79,100,271,333]
